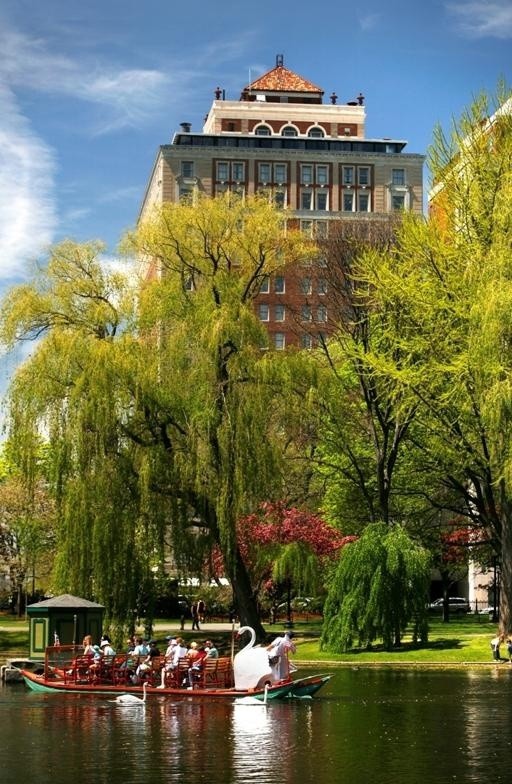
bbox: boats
[18,635,337,701]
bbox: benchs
[62,648,232,692]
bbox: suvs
[428,597,469,614]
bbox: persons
[197,599,206,622]
[506,640,512,663]
[190,601,200,630]
[67,635,218,689]
[266,630,295,665]
[490,633,505,660]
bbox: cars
[480,606,499,617]
[293,594,317,611]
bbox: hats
[171,639,177,645]
[100,640,111,647]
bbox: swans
[116,681,153,703]
